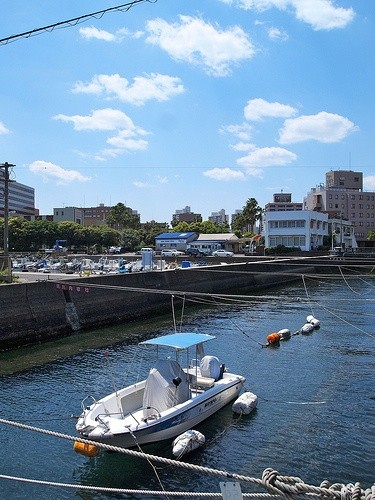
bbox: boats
[76,331,247,450]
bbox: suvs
[185,248,204,257]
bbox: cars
[212,249,234,257]
[8,245,141,275]
[161,249,178,257]
[177,251,185,255]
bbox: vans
[141,248,155,256]
